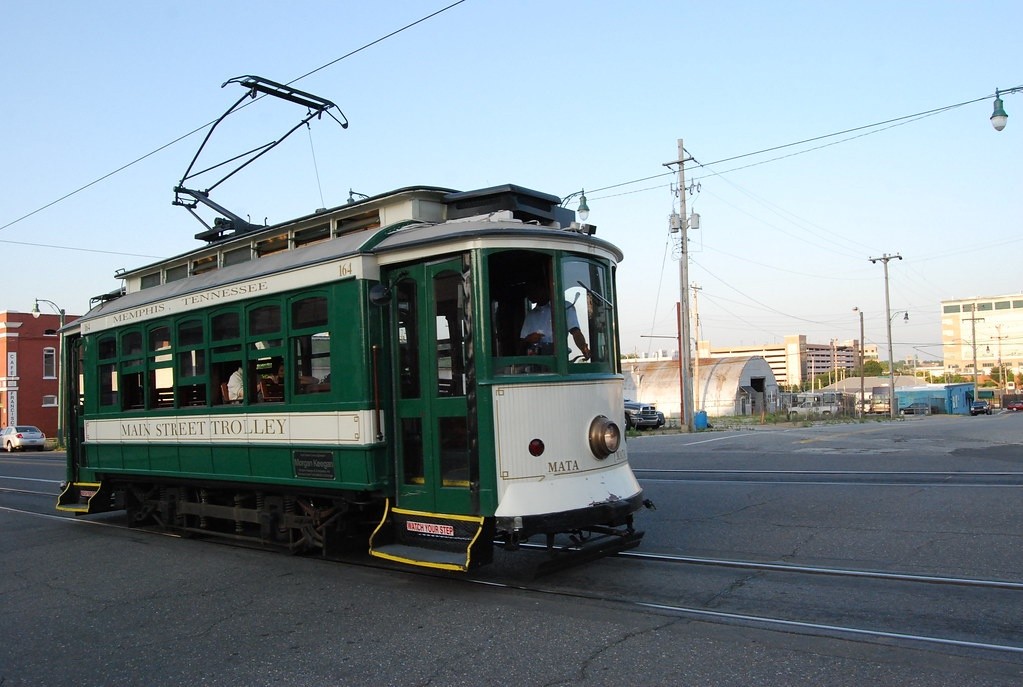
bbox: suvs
[970,399,993,416]
[1006,400,1023,412]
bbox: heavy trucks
[869,386,945,415]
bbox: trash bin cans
[695,411,707,430]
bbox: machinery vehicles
[813,392,844,419]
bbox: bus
[55,184,658,576]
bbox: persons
[227,362,279,405]
[277,361,319,391]
[520,279,590,372]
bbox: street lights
[851,305,865,419]
[31,297,65,449]
[868,252,909,420]
[952,303,990,402]
[991,322,1008,411]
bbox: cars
[855,400,872,414]
[1007,381,1016,390]
[787,402,819,420]
[623,396,666,430]
[0,425,47,453]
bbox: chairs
[220,379,285,401]
